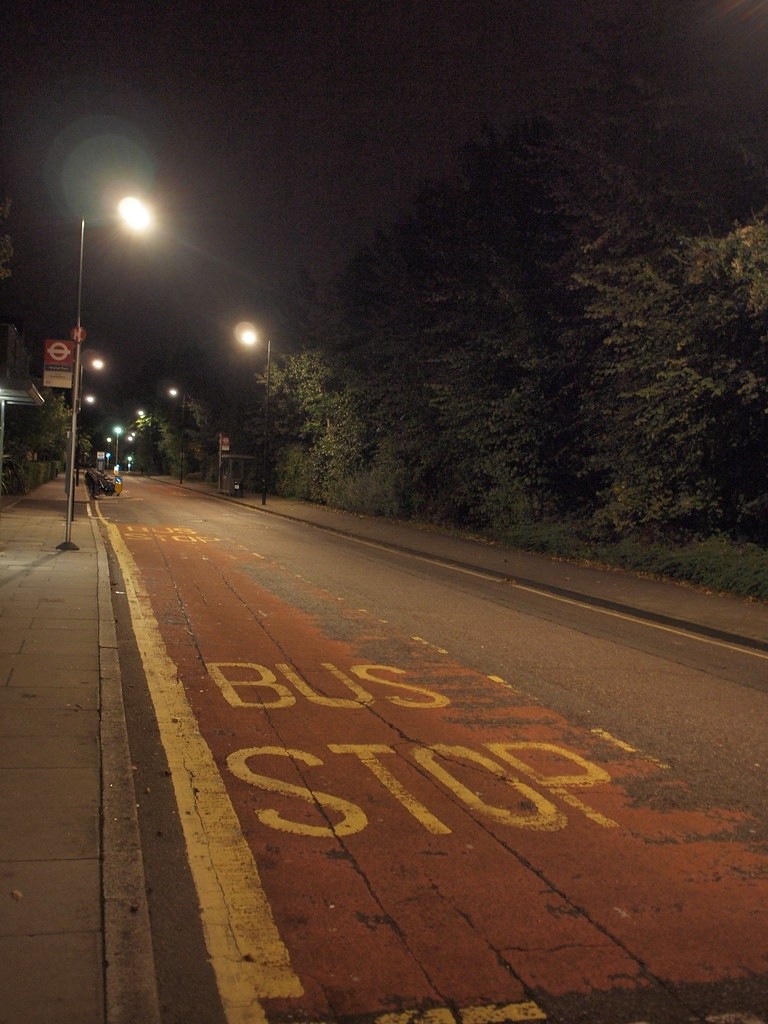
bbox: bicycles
[85,468,117,498]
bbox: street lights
[137,410,153,477]
[169,387,186,484]
[106,437,112,470]
[74,355,105,486]
[113,425,122,468]
[56,194,156,552]
[235,321,272,507]
